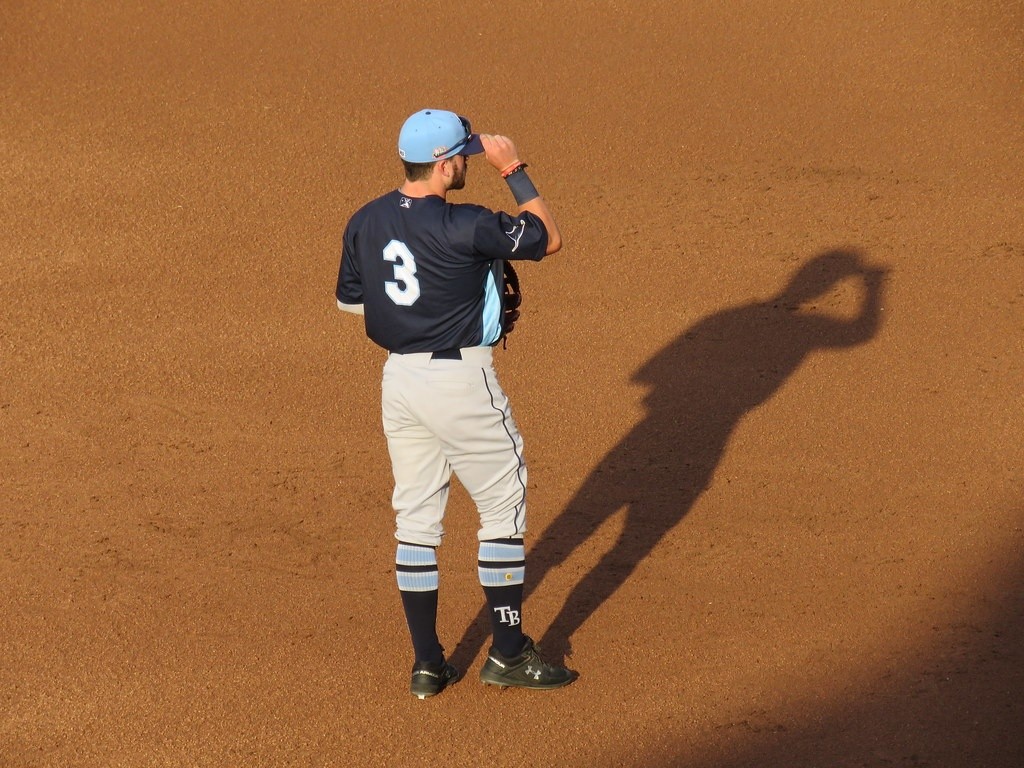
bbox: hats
[398,109,485,163]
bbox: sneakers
[480,634,577,690]
[410,643,459,700]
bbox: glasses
[434,116,472,158]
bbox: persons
[334,108,574,698]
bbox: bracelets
[499,161,523,178]
